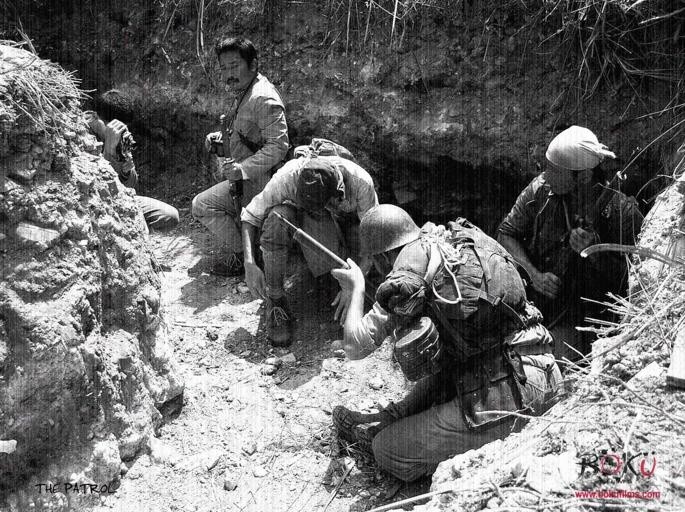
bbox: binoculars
[209,137,224,157]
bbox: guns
[211,112,242,232]
[272,210,377,307]
[535,146,643,308]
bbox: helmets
[357,203,422,258]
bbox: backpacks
[430,222,528,355]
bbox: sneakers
[266,298,294,348]
[333,406,391,452]
[209,251,248,277]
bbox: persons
[494,124,645,372]
[331,204,566,483]
[239,138,380,346]
[190,37,288,278]
[81,110,180,233]
